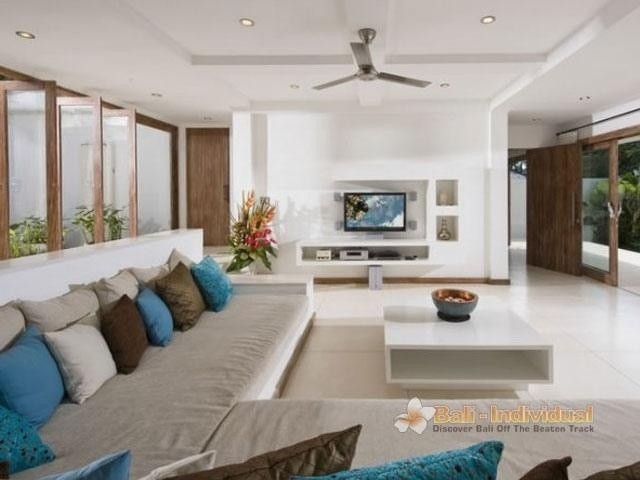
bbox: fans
[308,27,432,93]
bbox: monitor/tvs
[344,193,406,231]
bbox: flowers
[224,189,281,273]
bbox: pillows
[1,248,235,480]
[167,420,364,480]
[286,437,510,480]
[510,453,576,480]
[581,461,640,480]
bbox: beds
[381,302,558,394]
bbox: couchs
[1,226,317,480]
[200,397,639,480]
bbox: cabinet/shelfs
[294,237,436,293]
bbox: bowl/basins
[430,287,481,322]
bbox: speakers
[409,192,417,200]
[334,192,341,201]
[335,221,341,230]
[410,221,417,230]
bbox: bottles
[438,217,451,241]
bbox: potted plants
[15,215,65,254]
[75,206,126,245]
